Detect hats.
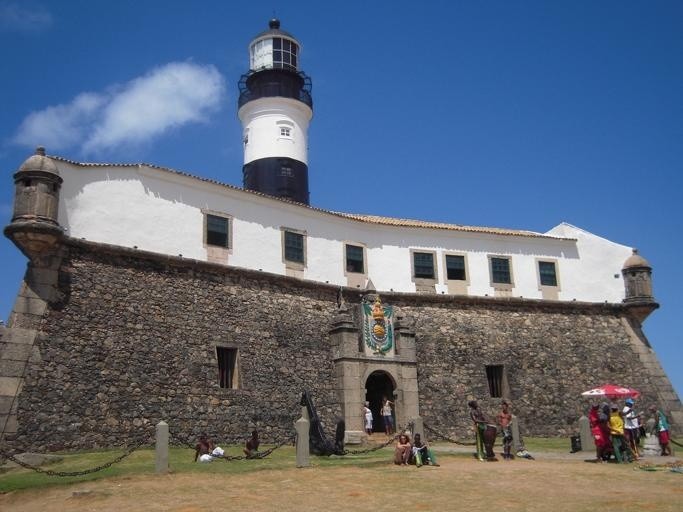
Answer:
[593,399,635,411]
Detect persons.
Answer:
[411,433,440,467]
[193,436,227,464]
[587,398,647,464]
[467,399,490,463]
[648,404,673,456]
[240,430,260,459]
[332,411,345,452]
[361,400,373,435]
[392,434,412,466]
[516,433,535,460]
[379,395,395,435]
[496,401,513,461]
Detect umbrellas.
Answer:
[579,382,641,402]
